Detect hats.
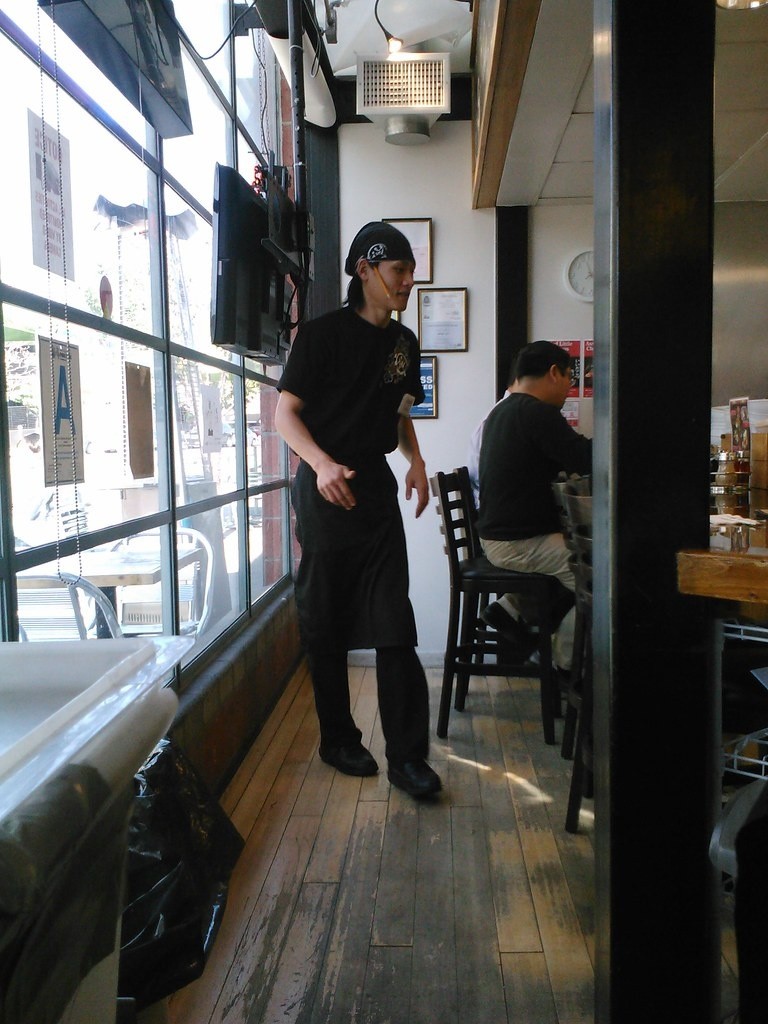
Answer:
[344,222,416,276]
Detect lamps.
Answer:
[375,0,403,53]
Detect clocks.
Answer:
[563,250,594,303]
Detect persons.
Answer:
[275,221,441,801]
[467,340,593,666]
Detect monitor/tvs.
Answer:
[209,163,291,367]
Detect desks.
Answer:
[20,545,203,641]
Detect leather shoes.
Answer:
[318,742,379,776]
[388,759,443,797]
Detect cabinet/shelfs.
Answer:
[712,605,768,955]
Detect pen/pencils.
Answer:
[373,266,391,298]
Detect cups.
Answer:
[710,525,732,554]
[731,525,750,553]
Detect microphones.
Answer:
[373,266,391,299]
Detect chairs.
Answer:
[18,526,215,641]
[557,475,595,835]
[428,468,564,742]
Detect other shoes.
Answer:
[483,601,536,649]
